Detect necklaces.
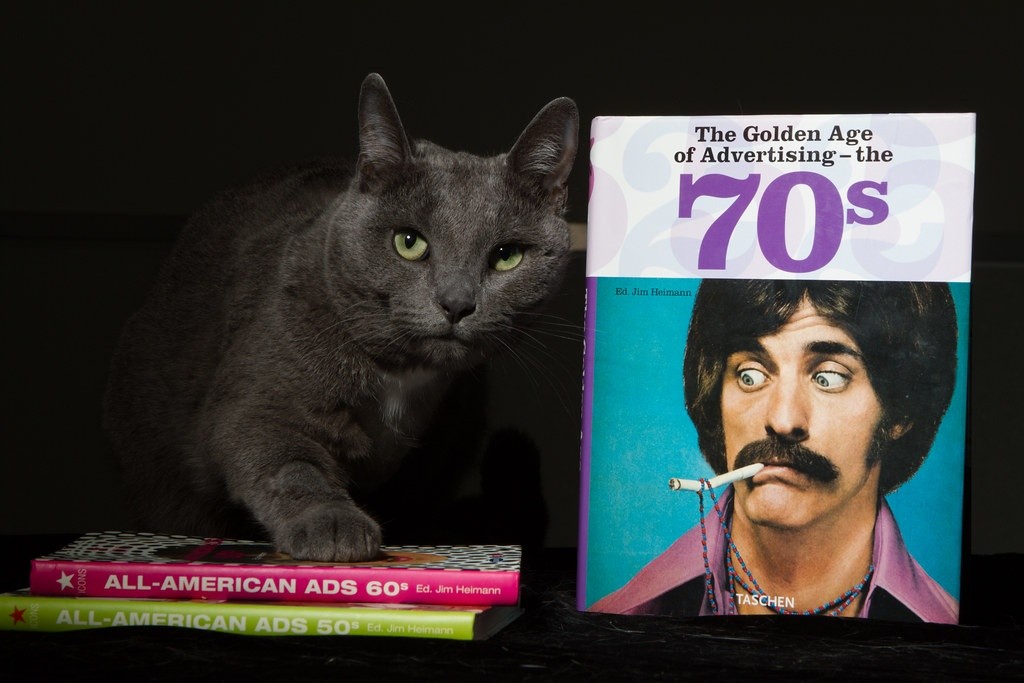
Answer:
[698,478,875,615]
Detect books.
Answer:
[576,112,976,624]
[29,526,521,605]
[0,587,525,642]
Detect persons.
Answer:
[590,279,957,624]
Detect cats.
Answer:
[0,70,579,563]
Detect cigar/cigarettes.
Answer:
[669,464,764,493]
